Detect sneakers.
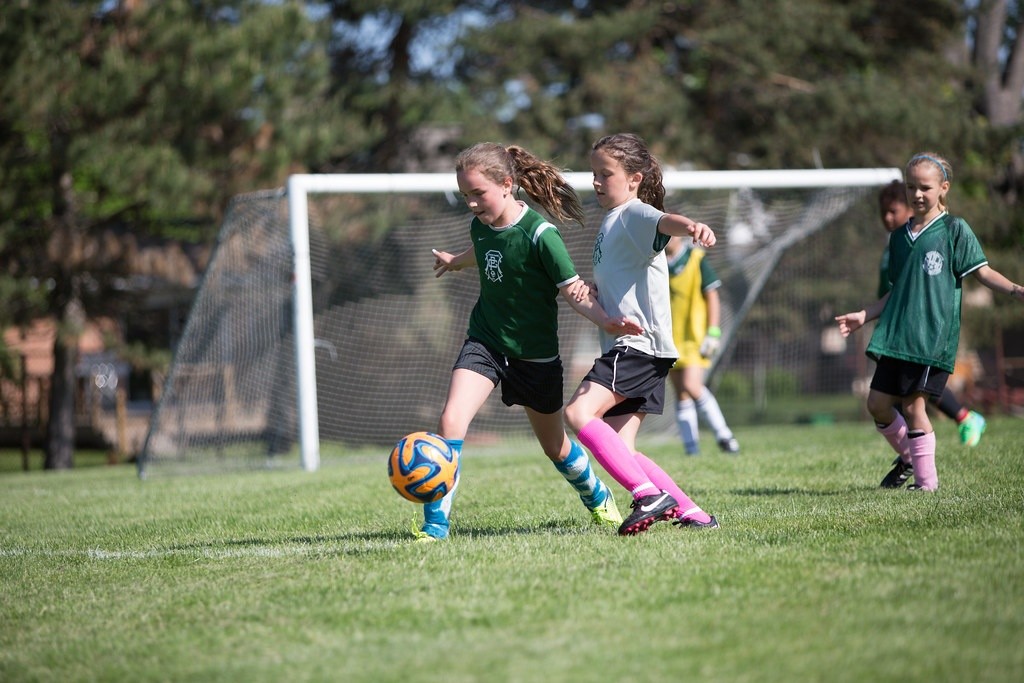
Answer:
[588,485,623,528]
[410,508,451,545]
[906,484,932,493]
[958,410,987,447]
[618,489,680,535]
[671,515,721,531]
[880,457,914,490]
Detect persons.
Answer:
[833,151,1024,490]
[875,178,986,448]
[407,141,646,543]
[661,232,740,458]
[561,133,722,536]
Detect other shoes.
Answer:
[718,436,740,453]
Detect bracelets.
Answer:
[709,326,721,336]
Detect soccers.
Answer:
[385,431,460,502]
